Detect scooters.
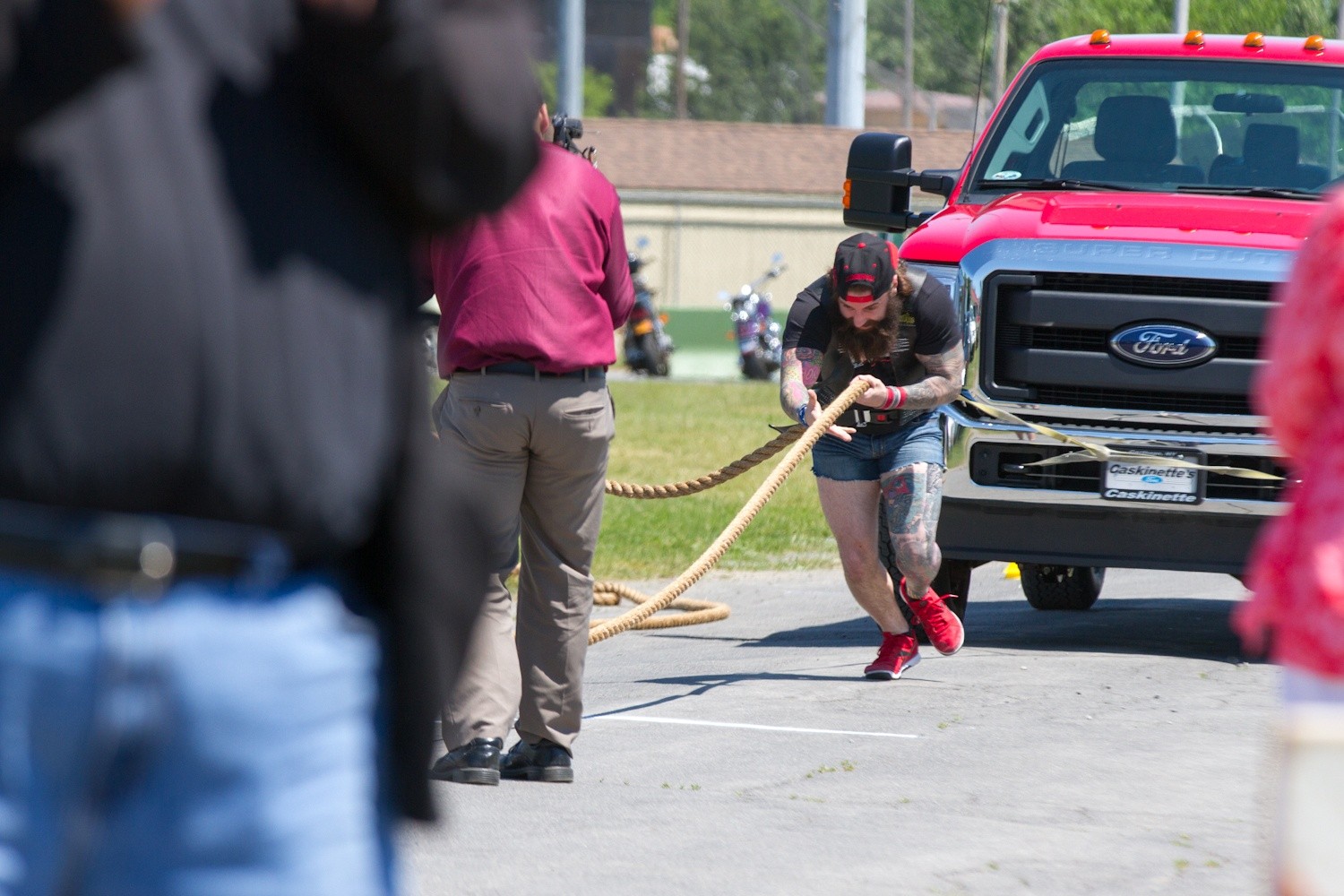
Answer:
[617,237,676,377]
[716,250,791,382]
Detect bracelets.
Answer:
[796,404,809,428]
[876,386,906,411]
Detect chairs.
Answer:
[1067,94,1204,184]
[1208,123,1329,189]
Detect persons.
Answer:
[779,233,965,680]
[1233,189,1344,895]
[427,105,636,784]
[0,1,542,896]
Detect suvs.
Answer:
[840,26,1344,645]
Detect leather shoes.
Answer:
[432,737,501,784]
[500,739,573,781]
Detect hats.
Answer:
[831,233,898,303]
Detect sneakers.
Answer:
[865,629,920,679]
[900,577,964,656]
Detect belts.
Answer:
[1,536,317,594]
[456,362,605,380]
